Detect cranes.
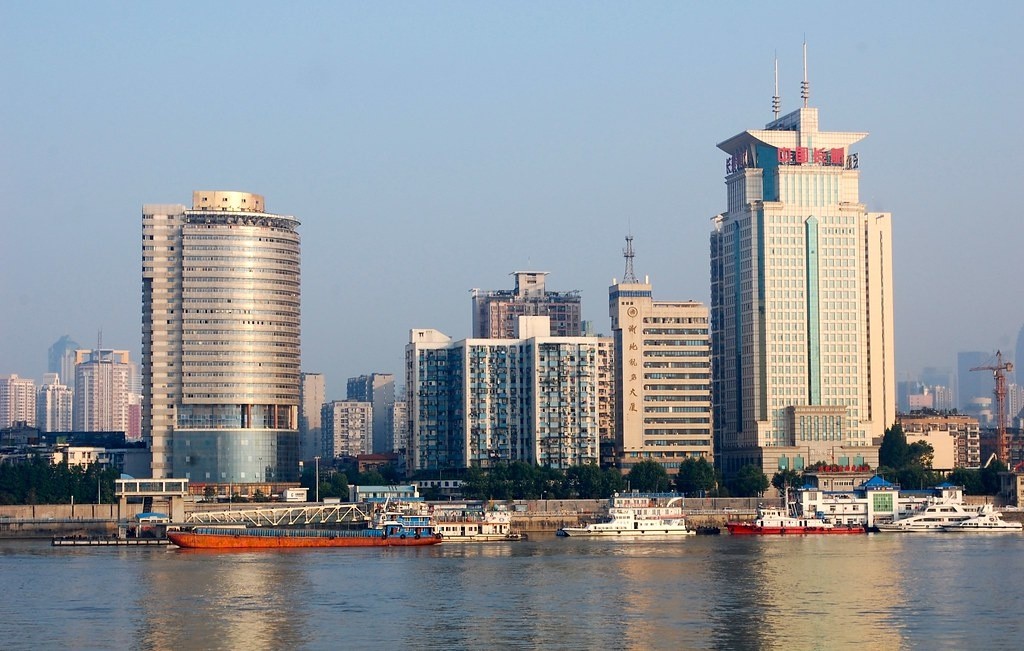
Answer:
[968,348,1015,467]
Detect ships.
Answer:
[722,483,867,535]
[871,502,981,533]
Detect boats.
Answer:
[555,514,696,537]
[171,502,446,547]
[940,503,1022,533]
[436,533,521,542]
